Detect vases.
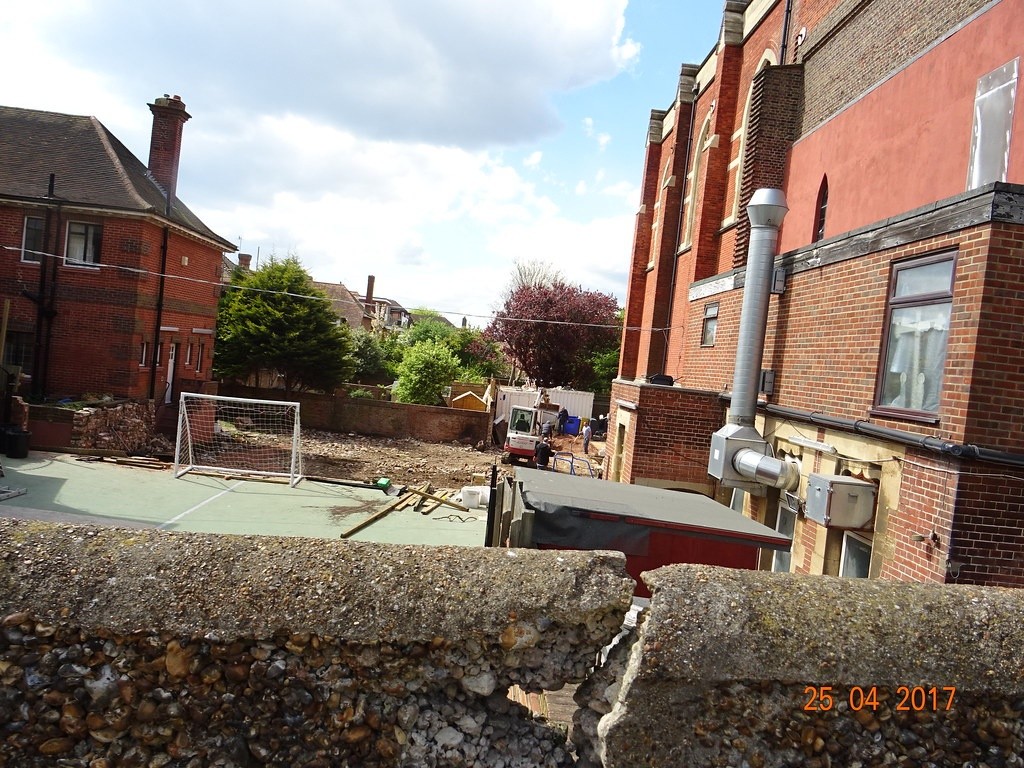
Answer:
[6,431,32,458]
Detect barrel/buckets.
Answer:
[478,486,490,504]
[461,485,480,509]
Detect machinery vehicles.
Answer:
[500,387,561,470]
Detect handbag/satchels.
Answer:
[533,456,539,462]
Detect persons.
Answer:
[579,421,591,454]
[534,438,556,470]
[556,407,570,436]
[515,414,530,433]
[542,421,553,440]
